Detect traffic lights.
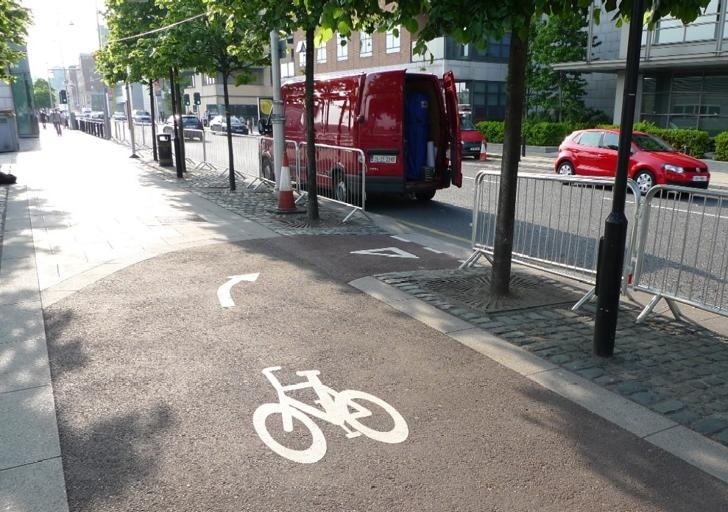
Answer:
[61,90,67,103]
[193,92,201,106]
[184,94,190,106]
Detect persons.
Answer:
[203,110,211,127]
[40,107,69,137]
[248,118,253,134]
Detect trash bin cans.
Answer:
[157,134,173,166]
[0,110,19,152]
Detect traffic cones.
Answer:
[479,139,488,161]
[264,152,308,214]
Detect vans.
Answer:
[257,68,462,200]
[457,116,486,158]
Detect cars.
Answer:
[554,127,709,197]
[114,111,127,120]
[162,114,205,140]
[132,109,152,127]
[209,115,249,135]
